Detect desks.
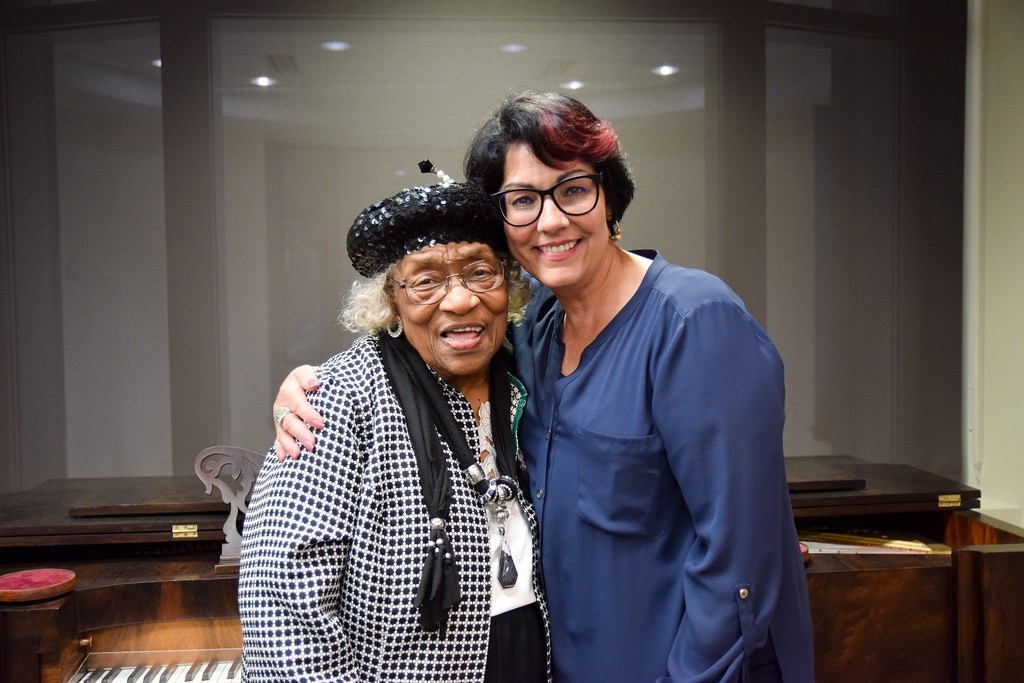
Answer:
[0,454,984,566]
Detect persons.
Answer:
[273,82,816,683]
[238,156,555,683]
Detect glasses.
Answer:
[390,259,507,305]
[491,171,604,227]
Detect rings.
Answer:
[276,406,292,427]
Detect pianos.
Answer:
[1,454,1023,683]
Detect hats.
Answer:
[346,158,510,279]
